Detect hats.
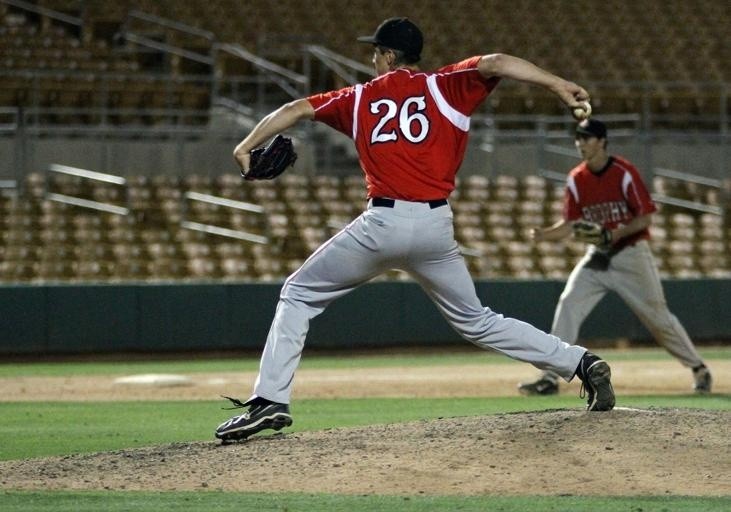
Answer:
[569,117,607,141]
[356,17,423,56]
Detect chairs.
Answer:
[0,0,731,280]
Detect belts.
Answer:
[372,198,448,209]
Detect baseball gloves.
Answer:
[241,134,297,181]
[572,218,613,247]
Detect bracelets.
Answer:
[601,227,612,245]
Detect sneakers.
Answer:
[518,376,559,396]
[215,394,294,441]
[576,351,616,412]
[691,364,714,391]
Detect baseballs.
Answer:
[574,100,591,118]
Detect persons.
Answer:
[215,16,617,445]
[515,117,714,396]
